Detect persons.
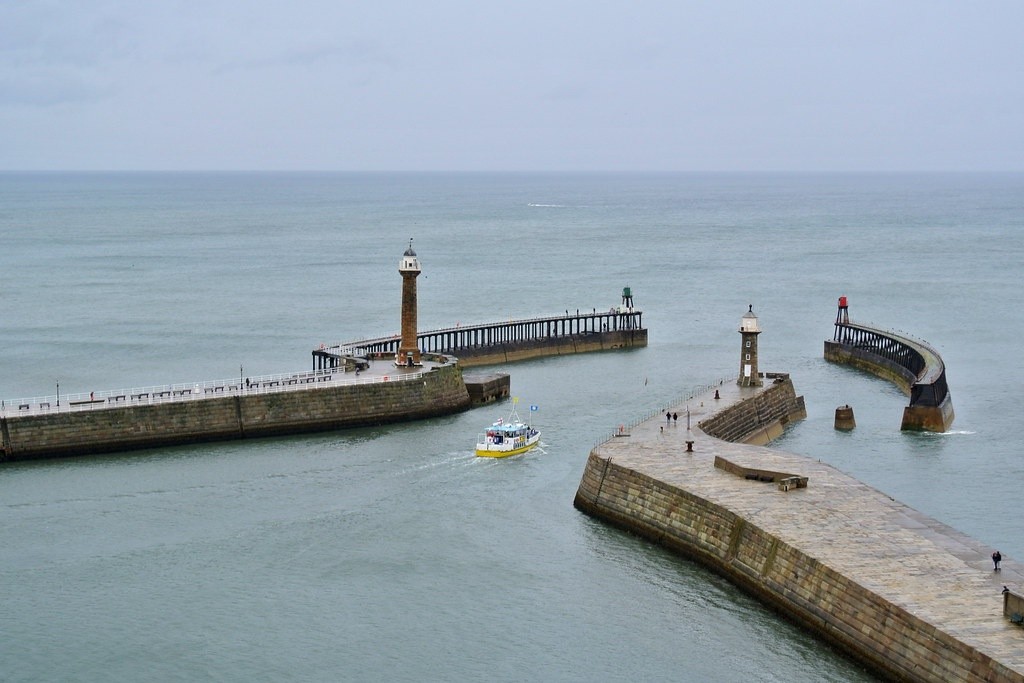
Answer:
[90,391,94,401]
[603,323,606,331]
[553,328,556,334]
[246,378,249,386]
[666,412,671,421]
[673,413,678,420]
[1,400,5,410]
[992,551,1002,571]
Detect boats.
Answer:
[475,397,541,458]
